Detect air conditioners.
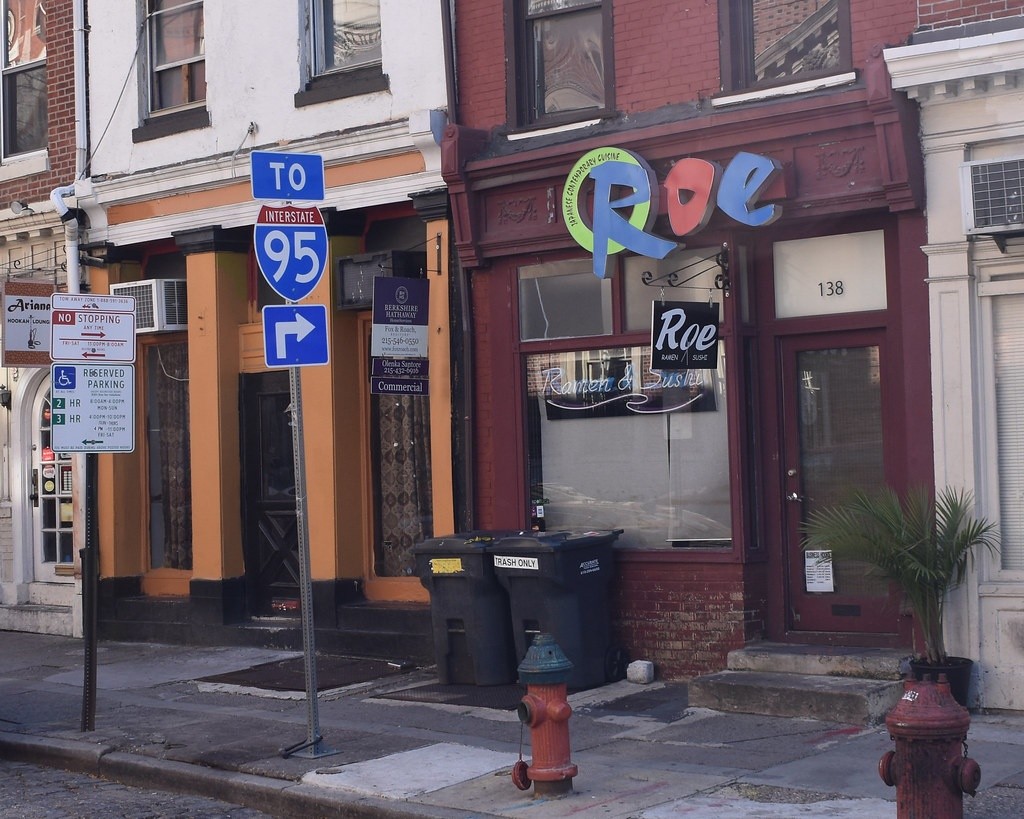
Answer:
[335,251,424,313]
[109,279,187,336]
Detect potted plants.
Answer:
[795,484,1011,706]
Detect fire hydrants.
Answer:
[511,633,579,798]
[878,673,982,818]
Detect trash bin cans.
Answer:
[409,530,515,690]
[487,526,632,693]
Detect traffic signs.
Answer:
[49,310,137,364]
[49,363,136,455]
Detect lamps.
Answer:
[0,384,12,408]
[11,199,36,214]
